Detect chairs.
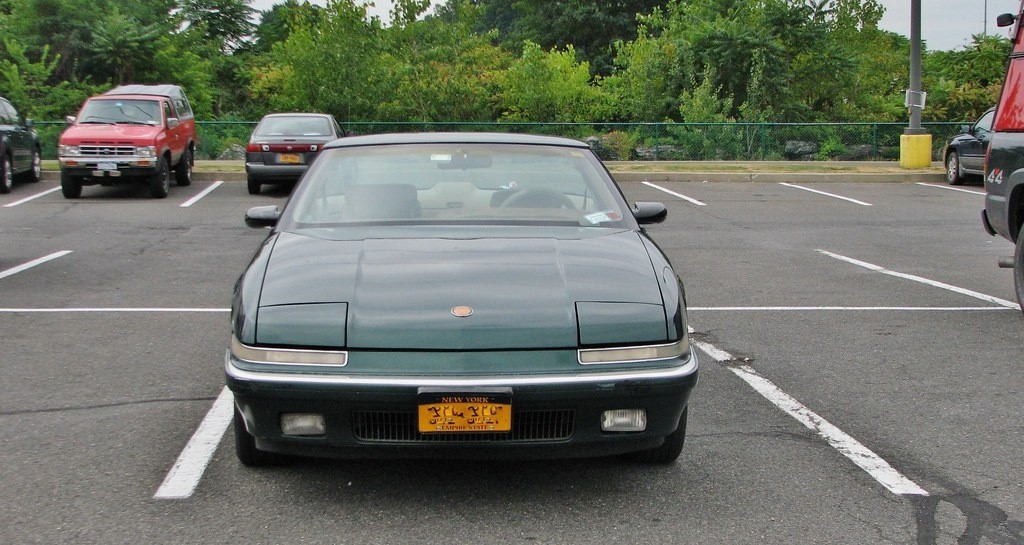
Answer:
[490,188,566,208]
[341,181,418,221]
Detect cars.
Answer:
[0,97,41,194]
[246,113,357,194]
[943,106,996,185]
[224,132,700,467]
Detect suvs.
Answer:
[58,85,197,198]
[980,1,1023,312]
